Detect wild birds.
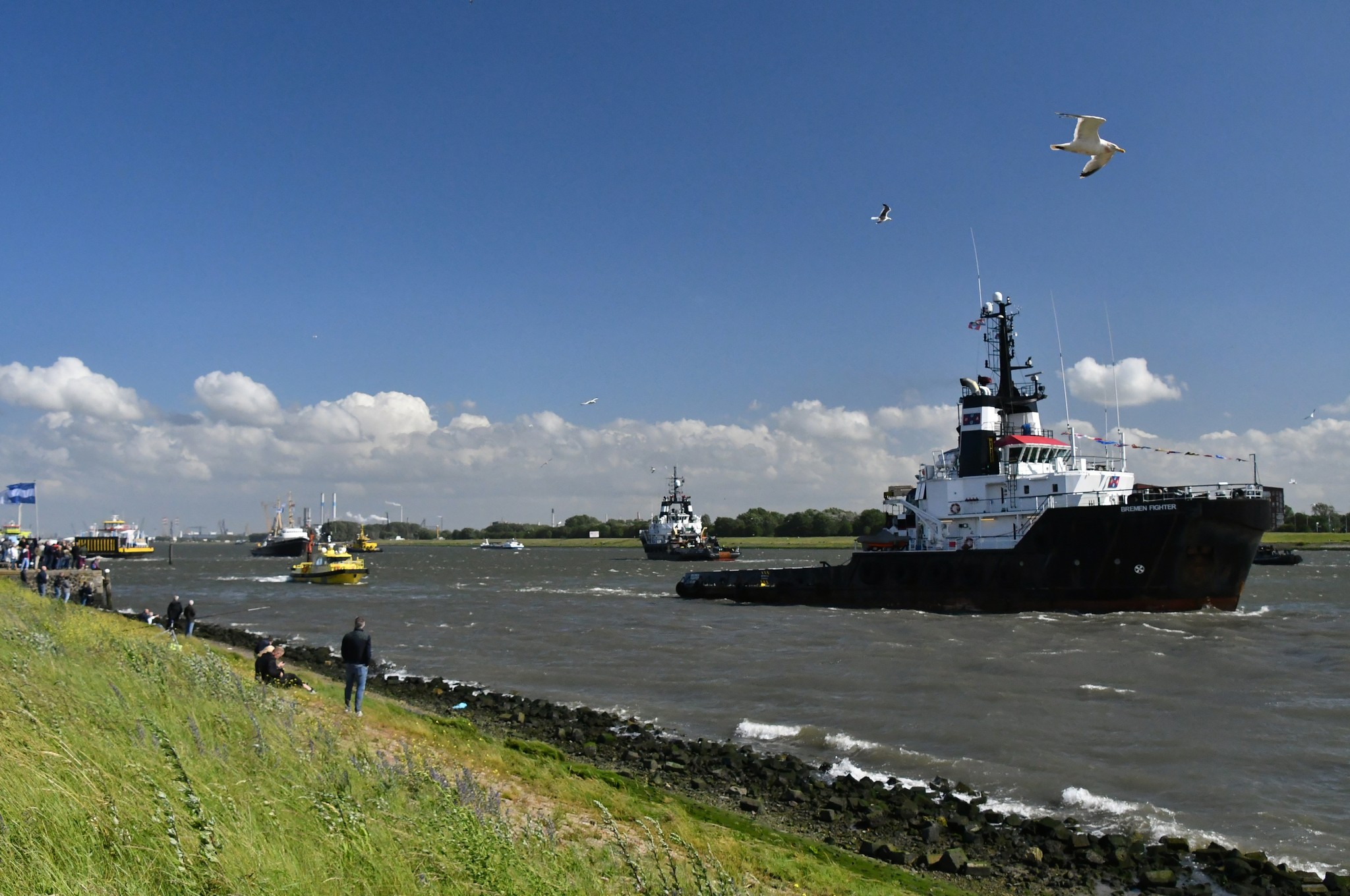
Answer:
[664,465,669,469]
[649,465,656,473]
[1303,408,1316,420]
[540,458,552,468]
[1049,110,1127,180]
[1288,478,1298,485]
[870,201,893,225]
[580,397,599,406]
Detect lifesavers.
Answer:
[965,538,974,548]
[950,503,961,514]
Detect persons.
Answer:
[80,581,93,606]
[37,566,49,598]
[20,567,28,588]
[140,608,161,624]
[91,555,106,579]
[184,599,197,637]
[166,595,183,630]
[341,616,373,718]
[254,637,320,695]
[0,537,39,571]
[42,540,88,570]
[62,576,73,603]
[53,572,64,600]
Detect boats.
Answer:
[674,283,1275,615]
[1252,544,1303,565]
[317,542,352,563]
[72,514,154,560]
[638,463,742,562]
[856,534,912,550]
[343,522,384,553]
[479,538,525,550]
[288,555,370,585]
[249,491,312,557]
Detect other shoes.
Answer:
[356,711,363,717]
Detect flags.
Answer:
[1061,432,1249,462]
[976,319,986,325]
[6,483,36,504]
[968,322,980,330]
[0,490,10,504]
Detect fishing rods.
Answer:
[122,606,270,632]
